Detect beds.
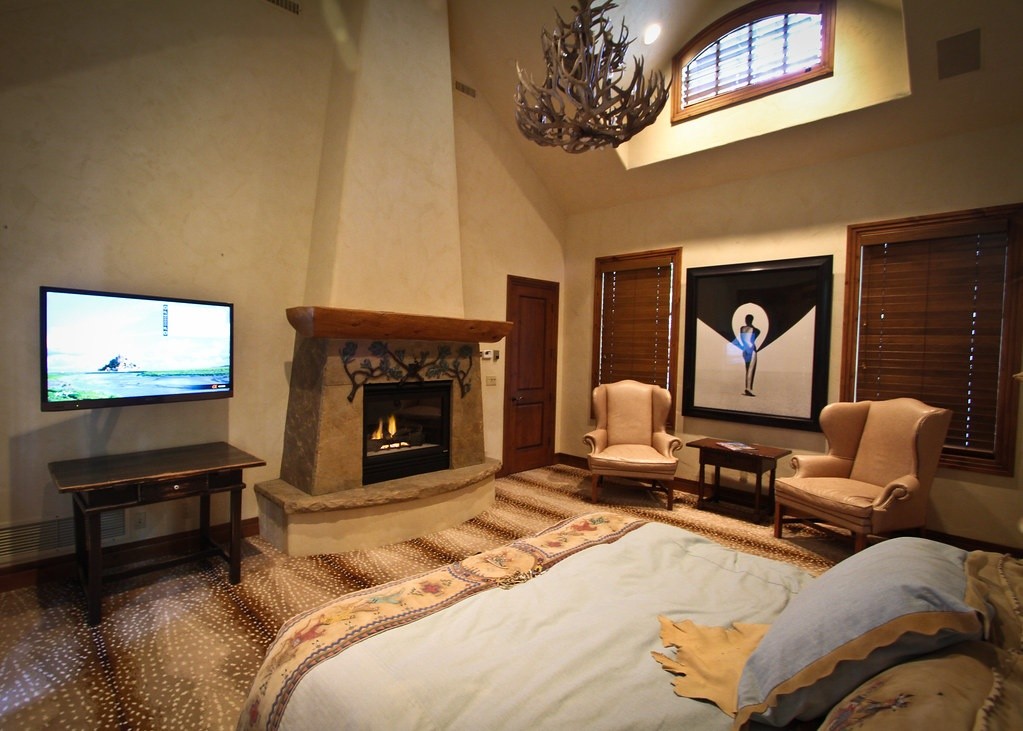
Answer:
[234,507,1023,731]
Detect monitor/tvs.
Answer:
[39,285,234,412]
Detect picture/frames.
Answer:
[681,251,836,431]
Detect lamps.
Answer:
[511,1,675,153]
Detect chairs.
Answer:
[774,395,951,557]
[580,379,680,512]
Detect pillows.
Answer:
[734,535,985,729]
[814,651,1005,731]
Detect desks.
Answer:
[48,441,267,628]
[685,436,792,524]
